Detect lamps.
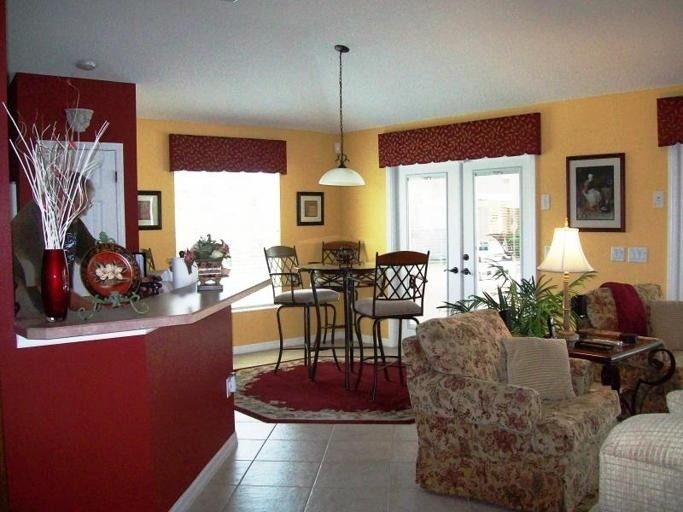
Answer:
[318,42,369,188]
[535,227,595,356]
[62,106,95,134]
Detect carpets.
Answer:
[231,356,416,425]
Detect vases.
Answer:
[39,248,70,324]
[192,257,225,291]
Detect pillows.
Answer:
[641,299,682,351]
[499,335,576,403]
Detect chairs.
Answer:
[599,381,683,511]
[352,247,437,399]
[140,247,155,274]
[317,236,366,376]
[400,306,624,512]
[570,279,682,415]
[261,245,346,383]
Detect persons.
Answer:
[10,171,162,312]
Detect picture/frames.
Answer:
[565,152,626,233]
[137,190,162,230]
[296,191,324,227]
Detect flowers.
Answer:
[182,233,228,276]
[89,259,130,286]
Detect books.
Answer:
[574,337,622,352]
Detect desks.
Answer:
[291,263,397,390]
[544,330,663,419]
[539,321,678,422]
